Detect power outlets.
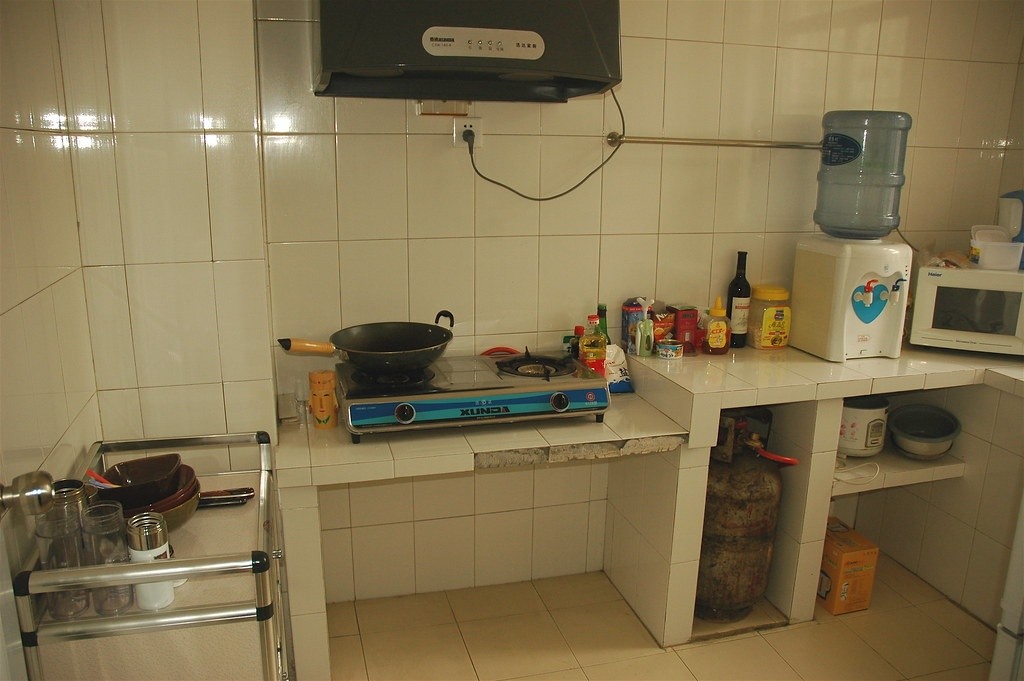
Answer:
[453,116,484,150]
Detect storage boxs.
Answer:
[969,241,1024,271]
[815,518,880,614]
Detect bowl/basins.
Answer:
[123,464,202,533]
[888,402,964,455]
[98,454,182,507]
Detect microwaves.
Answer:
[908,266,1024,356]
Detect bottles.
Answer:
[702,296,733,355]
[726,251,751,348]
[682,332,695,354]
[579,315,608,378]
[748,284,792,350]
[591,304,612,346]
[813,110,911,239]
[570,326,585,358]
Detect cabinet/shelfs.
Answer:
[12,430,291,681]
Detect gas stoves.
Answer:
[333,346,610,444]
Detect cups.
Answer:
[34,506,91,621]
[49,479,89,510]
[126,512,174,610]
[81,501,135,616]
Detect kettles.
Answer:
[998,188,1024,269]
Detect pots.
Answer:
[277,310,454,375]
[835,395,891,459]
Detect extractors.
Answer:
[308,0,622,104]
